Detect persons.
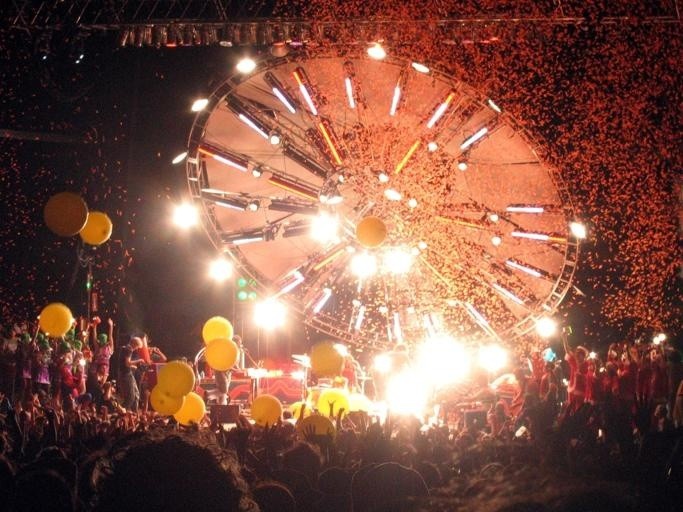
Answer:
[1,311,683,510]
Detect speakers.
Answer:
[464,409,492,432]
[208,404,243,423]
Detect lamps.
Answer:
[116,0,507,58]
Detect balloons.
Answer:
[354,217,387,245]
[39,192,349,441]
